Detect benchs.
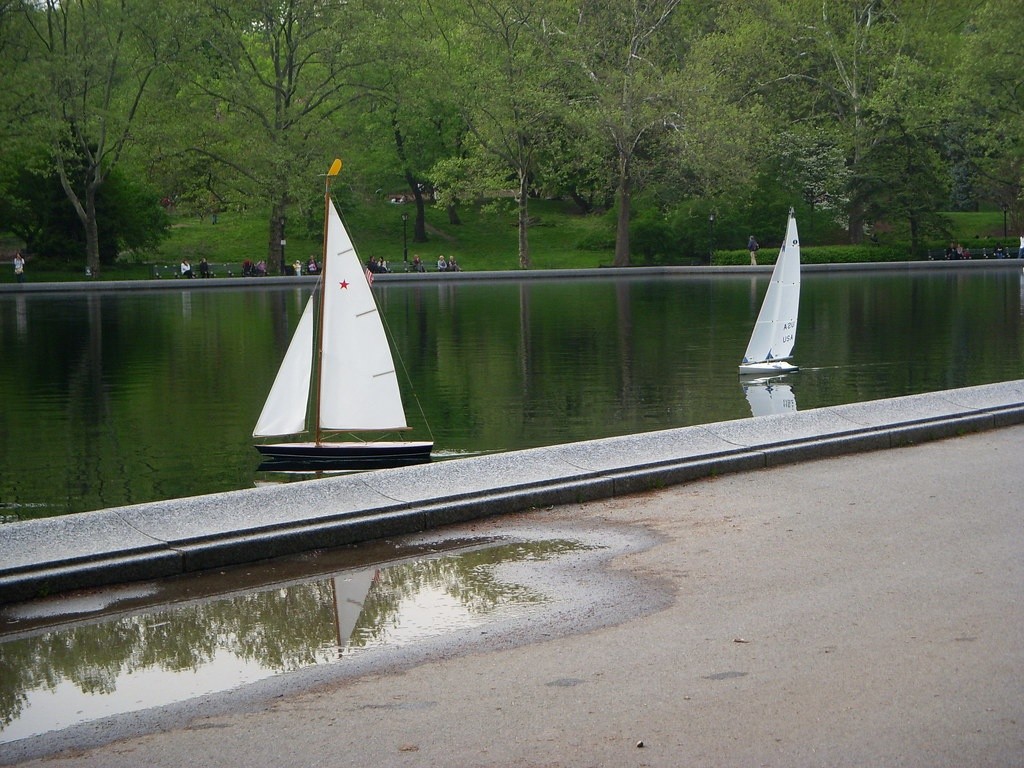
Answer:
[150,262,244,278]
[928,246,1019,261]
[285,264,323,276]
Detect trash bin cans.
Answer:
[285,265,294,275]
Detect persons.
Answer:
[946,233,1024,259]
[14,253,25,281]
[748,235,756,265]
[181,255,463,278]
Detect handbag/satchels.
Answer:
[15,268,23,274]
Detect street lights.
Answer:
[709,208,718,265]
[400,213,410,266]
[277,211,290,276]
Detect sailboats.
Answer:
[738,374,797,419]
[251,173,435,462]
[739,205,804,375]
[255,458,408,486]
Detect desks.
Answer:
[365,261,440,273]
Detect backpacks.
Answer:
[752,240,759,251]
